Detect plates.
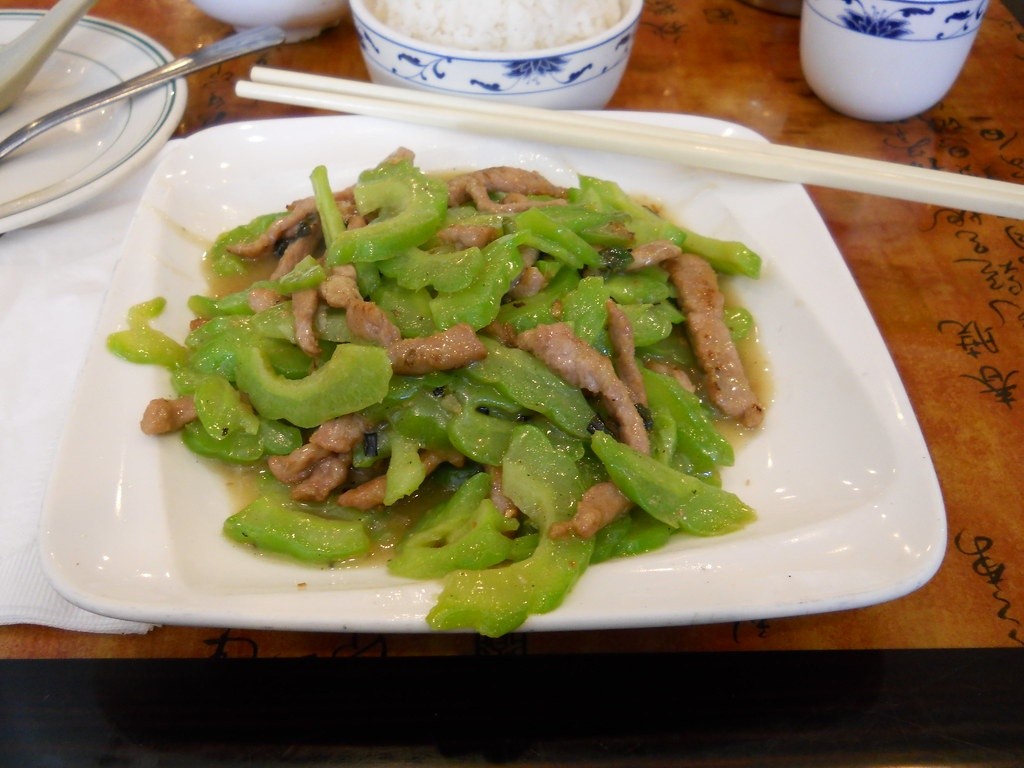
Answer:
[38,110,950,632]
[0,8,190,234]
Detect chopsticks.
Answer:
[235,65,1024,221]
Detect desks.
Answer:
[0,1,1024,765]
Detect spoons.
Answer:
[0,0,100,114]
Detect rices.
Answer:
[361,0,632,52]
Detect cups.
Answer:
[799,0,991,122]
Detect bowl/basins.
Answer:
[349,0,644,110]
[191,0,348,44]
